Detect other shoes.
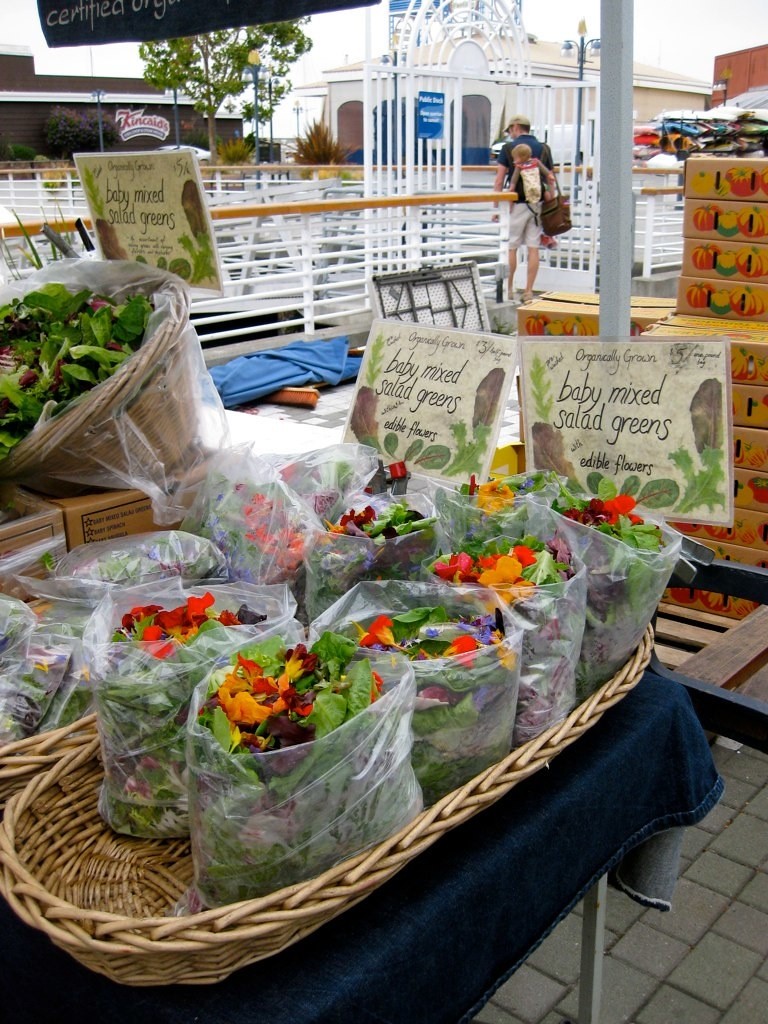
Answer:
[518,294,533,304]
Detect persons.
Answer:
[631,136,768,212]
[487,116,555,302]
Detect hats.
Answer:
[503,115,530,132]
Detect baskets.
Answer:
[0,266,198,496]
[0,620,655,987]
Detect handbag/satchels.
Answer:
[540,196,572,236]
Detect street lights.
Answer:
[268,77,280,181]
[561,19,602,206]
[292,101,303,138]
[242,51,268,188]
[92,88,107,152]
[381,51,398,192]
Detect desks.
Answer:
[0,642,726,1024]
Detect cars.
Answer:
[153,144,212,163]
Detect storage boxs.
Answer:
[40,456,210,550]
[514,150,768,625]
[0,478,68,604]
